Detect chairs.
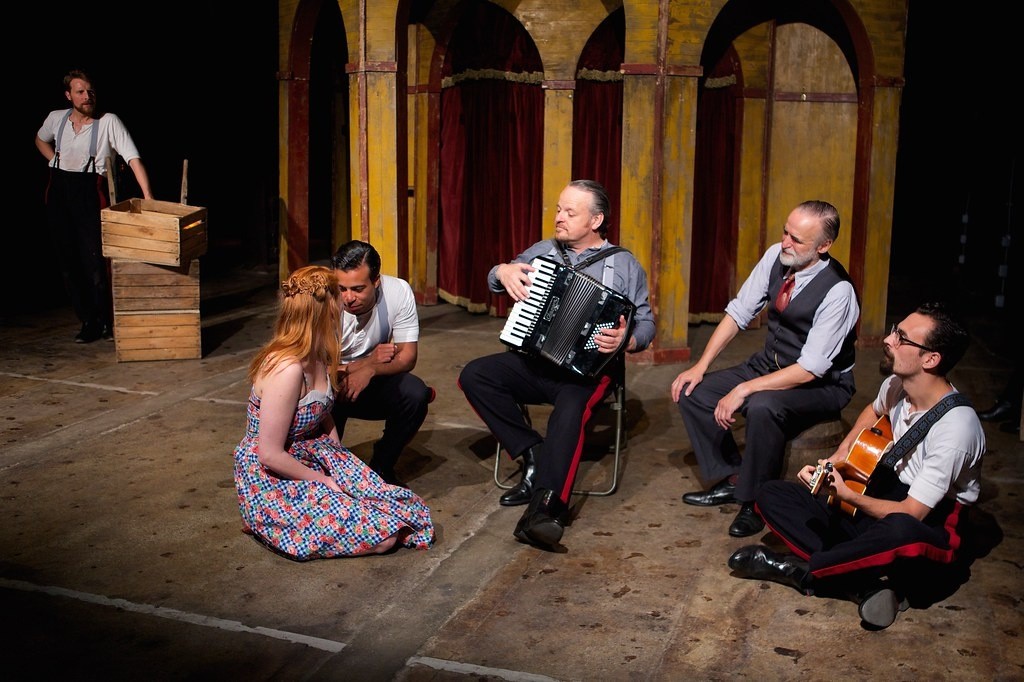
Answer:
[494,353,626,494]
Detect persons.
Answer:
[976,368,1024,434]
[670,200,860,536]
[330,240,429,489]
[457,180,656,547]
[725,299,988,630]
[35,70,154,343]
[232,265,435,562]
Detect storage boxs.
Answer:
[110,258,201,310]
[113,311,201,363]
[100,197,209,268]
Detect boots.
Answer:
[729,546,810,592]
[858,578,909,629]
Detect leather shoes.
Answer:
[977,399,1017,420]
[100,322,112,342]
[369,461,409,489]
[683,479,738,506]
[1000,418,1020,434]
[499,443,545,505]
[74,318,99,343]
[513,489,564,546]
[728,499,765,537]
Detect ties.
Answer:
[776,274,795,312]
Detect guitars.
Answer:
[806,412,897,519]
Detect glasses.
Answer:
[891,323,935,351]
